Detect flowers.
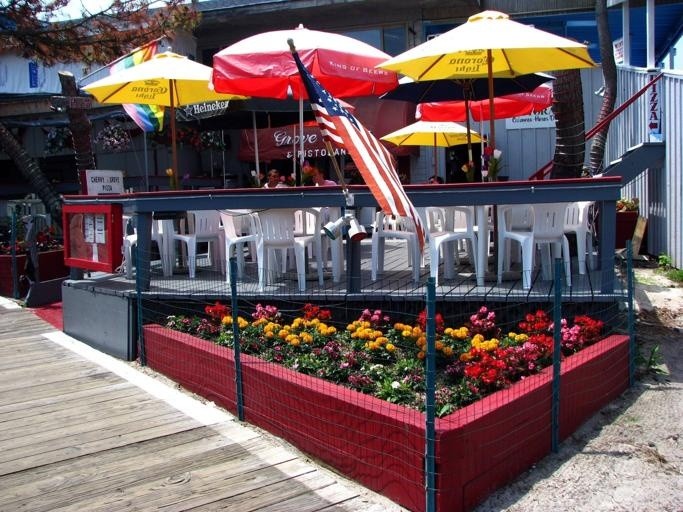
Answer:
[459,145,505,183]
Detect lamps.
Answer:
[323,187,374,250]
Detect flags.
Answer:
[290,53,425,255]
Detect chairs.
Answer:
[121,198,598,293]
